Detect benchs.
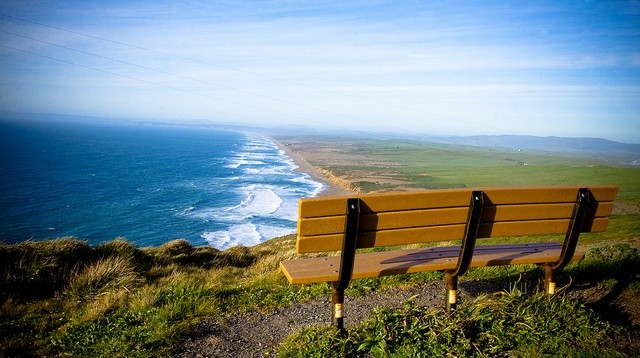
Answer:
[278,186,619,330]
